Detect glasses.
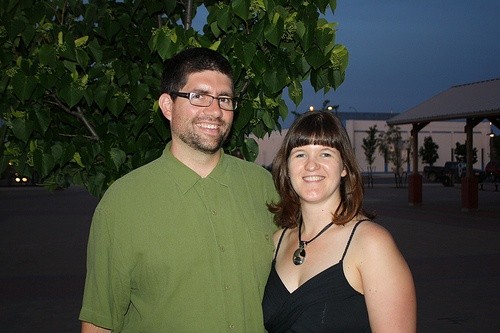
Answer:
[169,91,239,111]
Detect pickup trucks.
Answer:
[423,162,486,183]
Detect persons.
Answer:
[261,109,417,333]
[79,47,283,333]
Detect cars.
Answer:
[485,161,500,182]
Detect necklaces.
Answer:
[292,198,343,266]
